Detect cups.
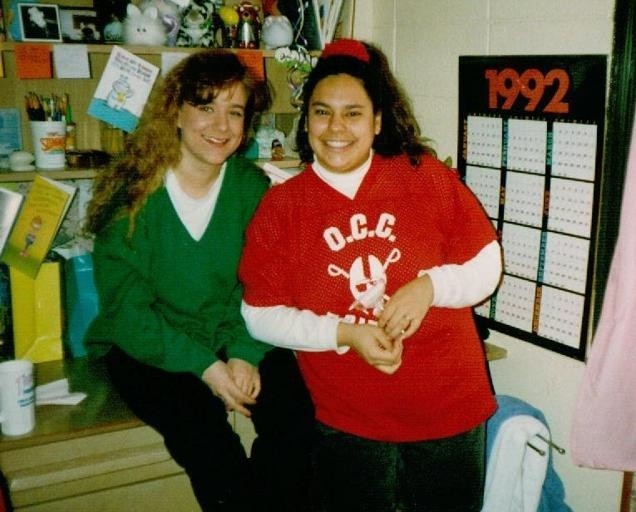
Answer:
[27,119,68,170]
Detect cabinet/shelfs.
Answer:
[0,0,505,512]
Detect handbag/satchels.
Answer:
[9,233,101,364]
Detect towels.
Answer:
[569,116,636,472]
[481,395,576,512]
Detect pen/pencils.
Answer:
[25,91,72,125]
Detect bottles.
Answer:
[0,361,36,436]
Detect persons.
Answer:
[83,49,321,508]
[238,38,503,512]
